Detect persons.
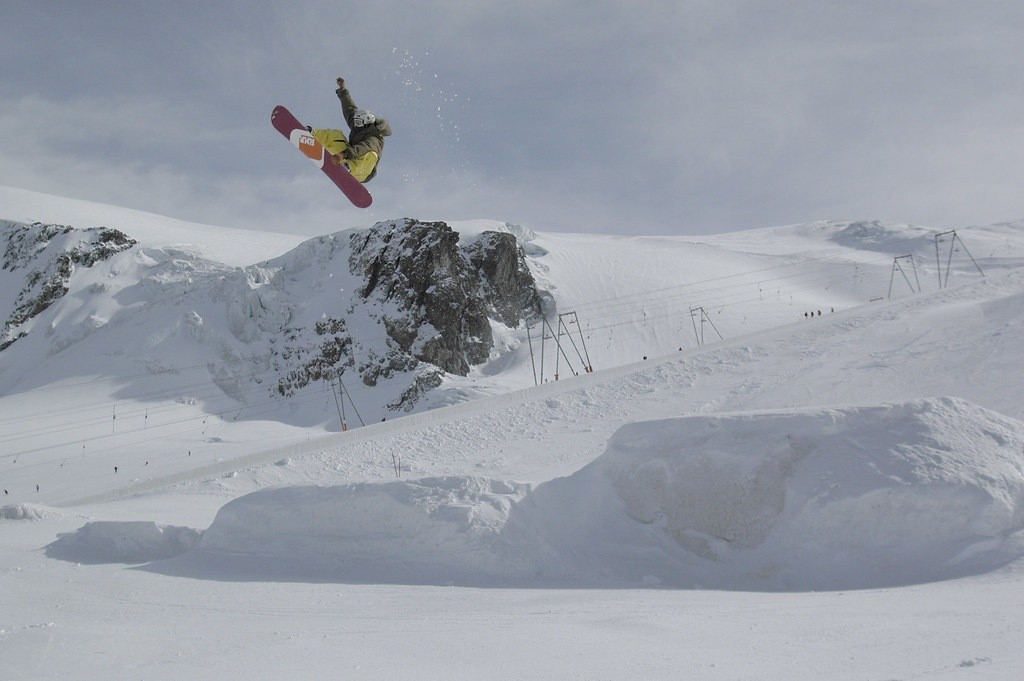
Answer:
[304,76,385,183]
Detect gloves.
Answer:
[331,151,347,165]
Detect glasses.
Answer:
[354,119,364,128]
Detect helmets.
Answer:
[353,111,376,129]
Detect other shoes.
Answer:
[343,164,350,172]
[305,125,312,133]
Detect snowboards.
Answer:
[270,105,374,209]
